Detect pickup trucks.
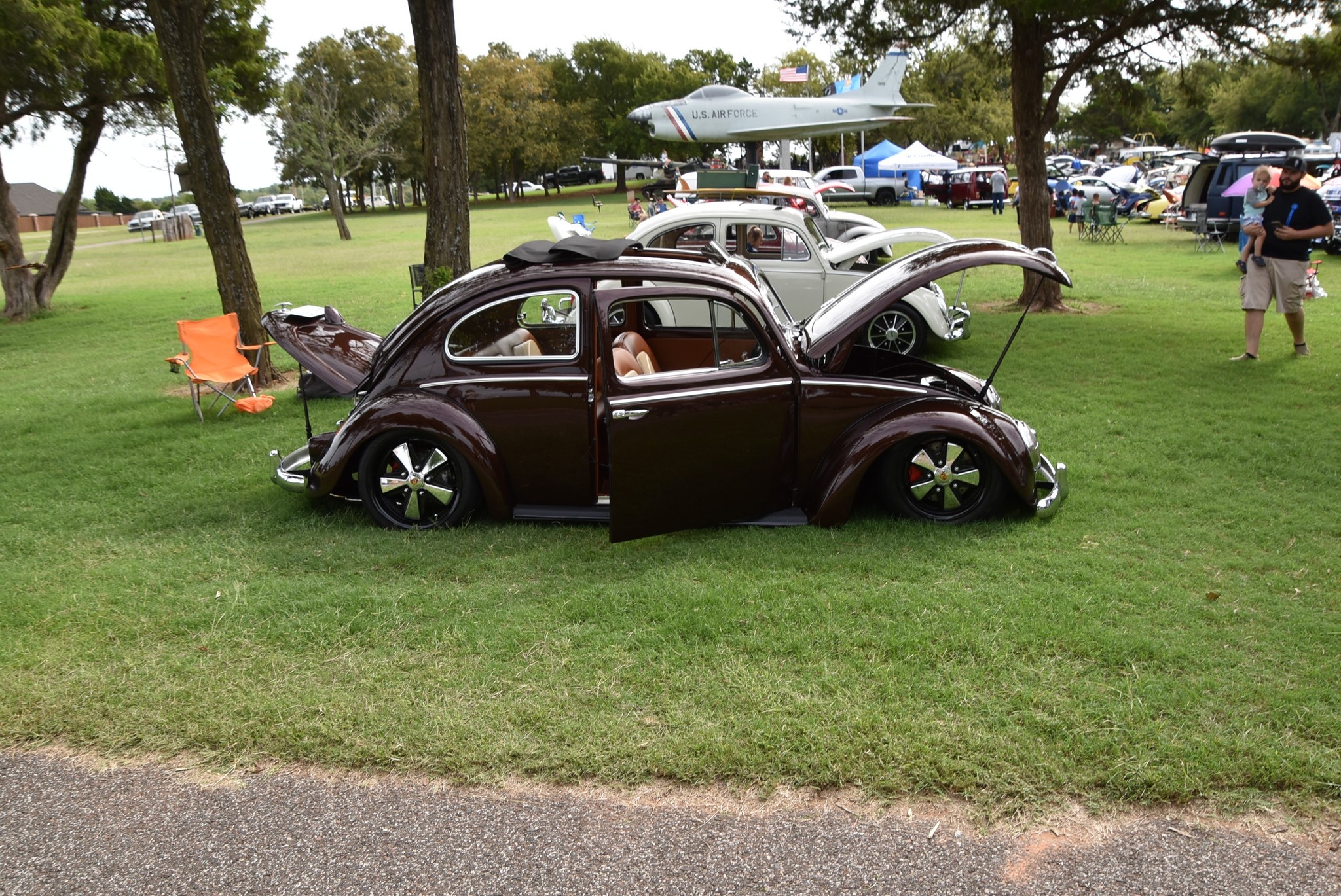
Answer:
[541,165,605,190]
[802,166,908,208]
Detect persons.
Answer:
[784,177,796,207]
[1046,186,1053,219]
[1334,152,1341,159]
[1153,140,1190,150]
[1044,145,1086,159]
[1132,162,1139,184]
[1068,189,1101,234]
[661,150,671,169]
[1228,156,1332,362]
[919,169,952,196]
[748,226,764,253]
[1012,186,1021,233]
[630,197,668,225]
[989,169,1007,215]
[902,172,917,198]
[1236,165,1276,273]
[1095,163,1105,177]
[1071,157,1082,171]
[674,166,682,186]
[754,172,773,204]
[1174,156,1182,162]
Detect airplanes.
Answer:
[626,41,936,143]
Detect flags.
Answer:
[779,65,809,82]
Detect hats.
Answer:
[1277,156,1307,172]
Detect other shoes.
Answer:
[1235,260,1247,274]
[1293,342,1310,357]
[1251,255,1266,268]
[1228,353,1260,361]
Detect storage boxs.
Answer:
[928,199,941,206]
[911,199,926,207]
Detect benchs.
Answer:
[592,194,603,213]
[453,327,545,356]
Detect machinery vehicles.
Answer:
[578,155,712,200]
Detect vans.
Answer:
[947,166,1011,210]
[758,170,836,204]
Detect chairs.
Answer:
[1158,200,1182,234]
[611,347,645,378]
[1090,199,1127,246]
[1079,199,1112,242]
[612,331,662,375]
[628,200,661,229]
[1306,260,1322,288]
[1192,210,1232,253]
[573,214,597,232]
[408,264,424,311]
[162,310,278,423]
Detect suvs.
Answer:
[321,190,359,211]
[364,195,396,208]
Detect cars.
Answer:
[127,193,304,233]
[540,181,972,356]
[501,181,543,194]
[1046,131,1341,255]
[260,235,1072,545]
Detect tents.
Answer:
[855,139,921,202]
[878,140,958,201]
